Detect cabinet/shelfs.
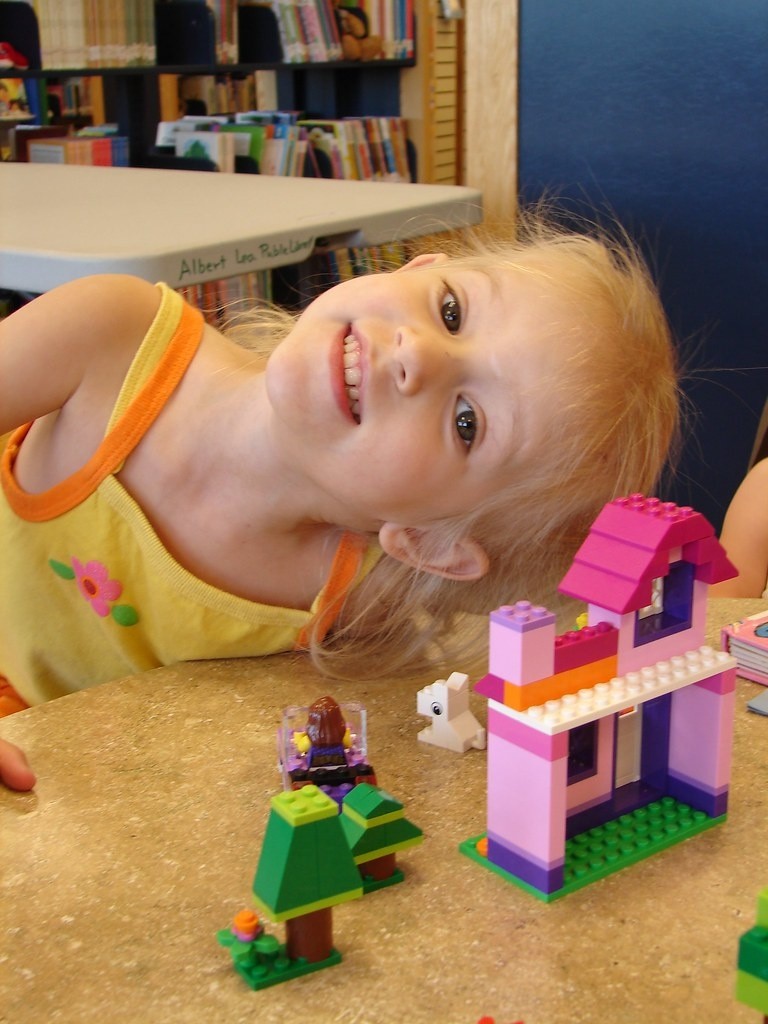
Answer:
[0,0,418,317]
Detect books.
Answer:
[0,0,418,332]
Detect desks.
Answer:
[0,161,485,294]
[0,597,768,1024]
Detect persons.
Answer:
[707,456,768,598]
[0,231,677,792]
